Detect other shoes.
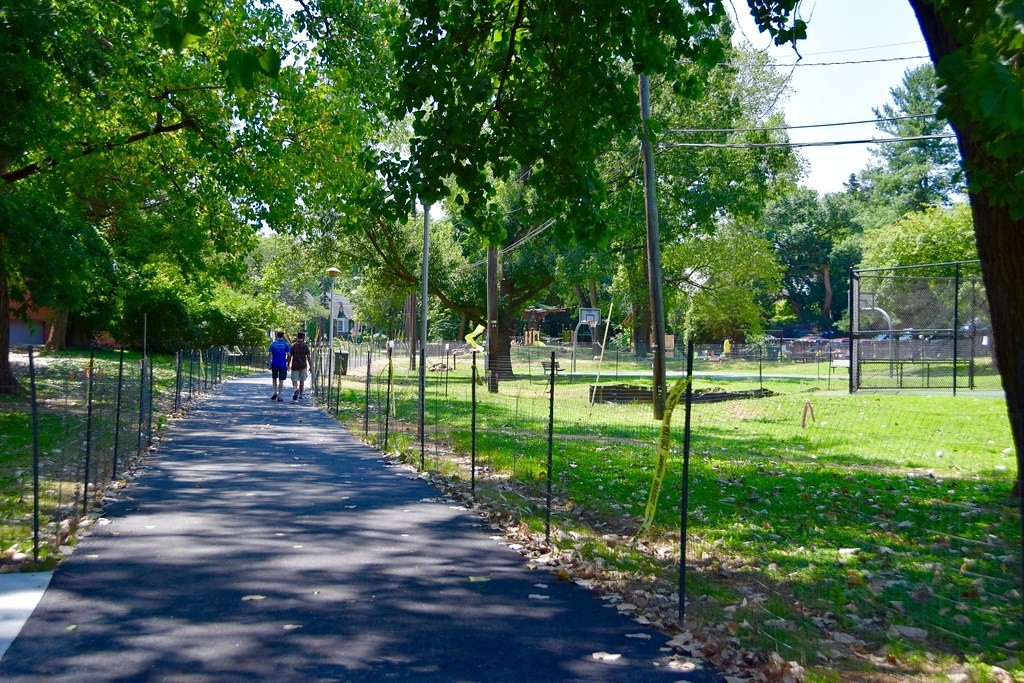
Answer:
[297,396,302,401]
[270,393,278,400]
[277,396,284,401]
[293,390,299,400]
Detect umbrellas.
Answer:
[796,336,878,344]
[745,334,778,342]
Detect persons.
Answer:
[290,333,314,400]
[724,337,732,353]
[809,344,814,352]
[268,331,291,401]
[386,338,394,357]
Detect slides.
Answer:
[464,324,486,352]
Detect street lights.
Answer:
[326,267,342,407]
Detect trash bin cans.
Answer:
[334,350,350,375]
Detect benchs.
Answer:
[542,361,566,374]
